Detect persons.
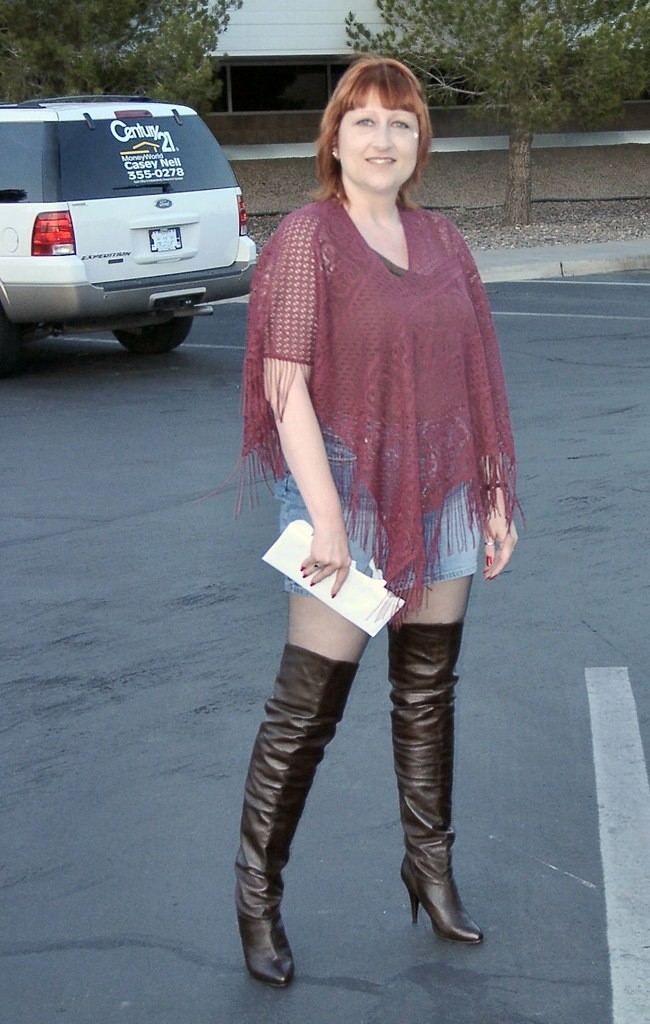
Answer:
[233,54,524,990]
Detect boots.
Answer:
[235,643,361,986]
[388,618,484,943]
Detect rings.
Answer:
[484,542,496,546]
[313,564,323,570]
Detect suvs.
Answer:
[0,94,259,377]
